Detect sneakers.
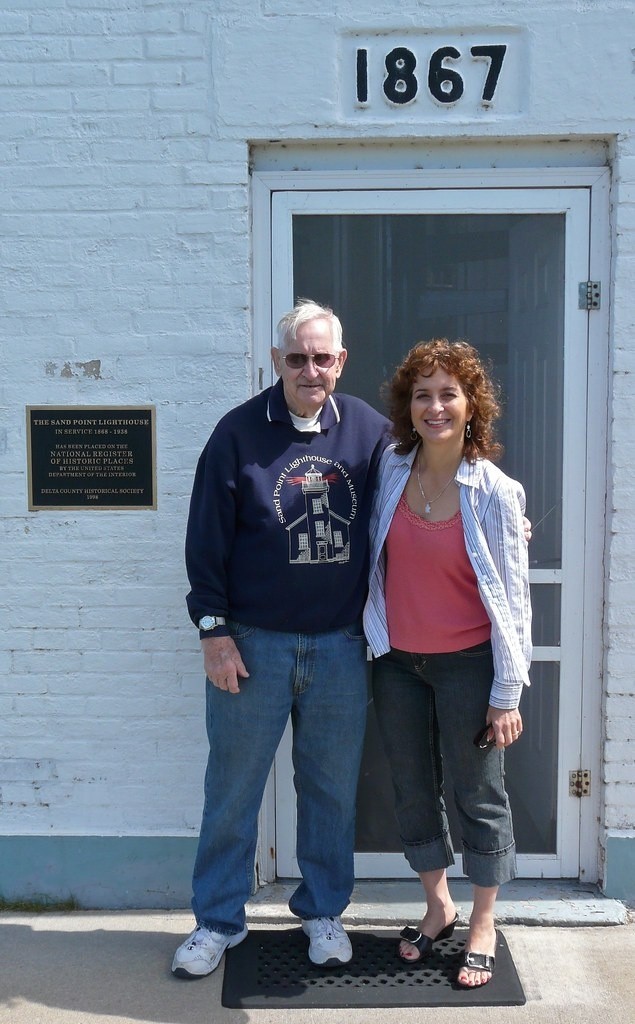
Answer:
[172,921,248,981]
[302,913,353,966]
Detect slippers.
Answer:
[456,928,498,988]
[399,911,459,963]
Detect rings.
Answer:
[512,731,519,736]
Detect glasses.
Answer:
[279,353,339,368]
[473,722,496,747]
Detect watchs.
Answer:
[199,616,225,632]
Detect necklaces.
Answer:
[417,453,455,514]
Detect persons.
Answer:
[172,304,532,980]
[362,340,534,989]
